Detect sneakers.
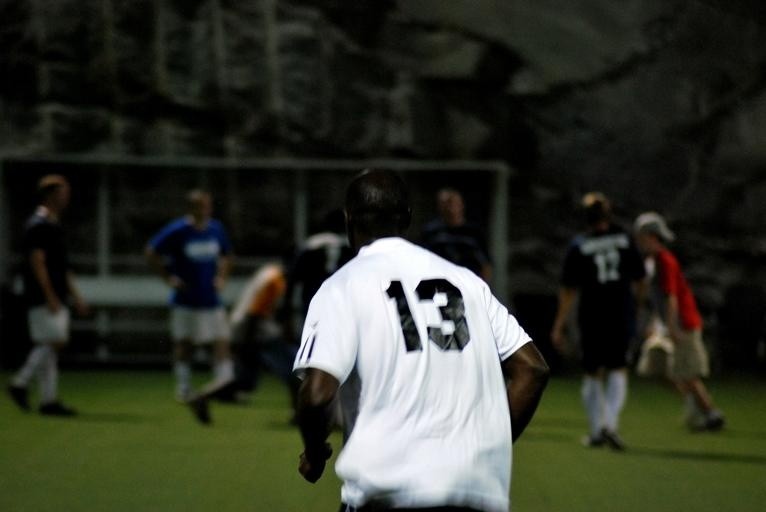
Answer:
[174,383,209,423]
[582,423,624,451]
[688,410,723,432]
[7,383,72,415]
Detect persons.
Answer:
[145,187,234,406]
[6,175,84,416]
[186,170,550,510]
[551,191,725,448]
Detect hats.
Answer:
[631,210,679,248]
